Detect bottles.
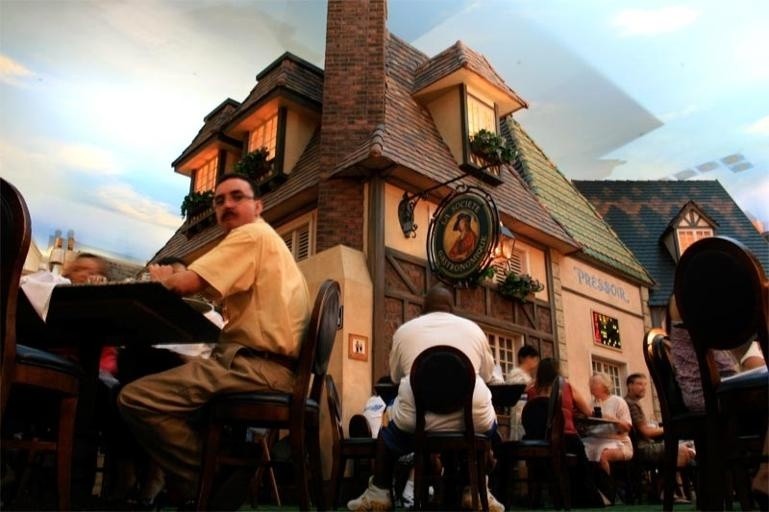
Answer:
[48,237,74,277]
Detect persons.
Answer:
[18,174,767,510]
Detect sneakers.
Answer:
[462,474,507,511]
[204,439,265,512]
[347,475,396,512]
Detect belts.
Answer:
[234,347,302,373]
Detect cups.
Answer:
[595,398,602,406]
[142,272,151,281]
[593,406,602,417]
[87,275,106,285]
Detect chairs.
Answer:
[0,178,85,512]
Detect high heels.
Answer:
[130,475,170,512]
